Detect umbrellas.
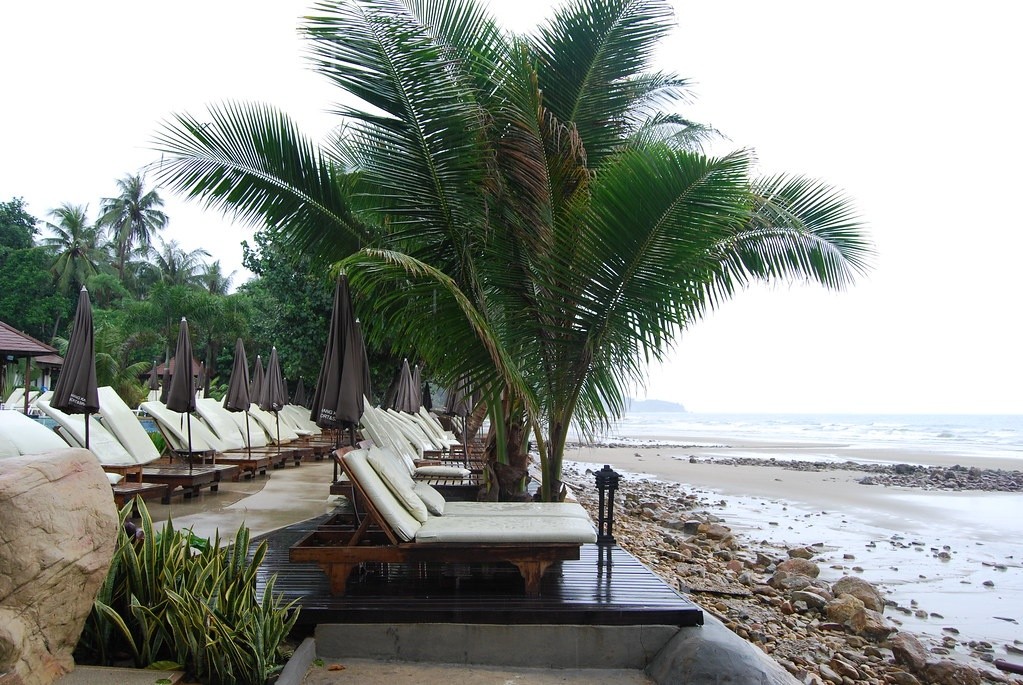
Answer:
[294,376,305,407]
[49,285,99,448]
[310,268,372,485]
[166,317,196,470]
[250,346,289,452]
[423,383,431,411]
[196,362,204,398]
[222,337,251,453]
[149,361,159,401]
[160,347,169,404]
[444,370,483,469]
[382,358,423,413]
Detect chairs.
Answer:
[0,386,599,596]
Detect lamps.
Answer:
[594,464,622,492]
